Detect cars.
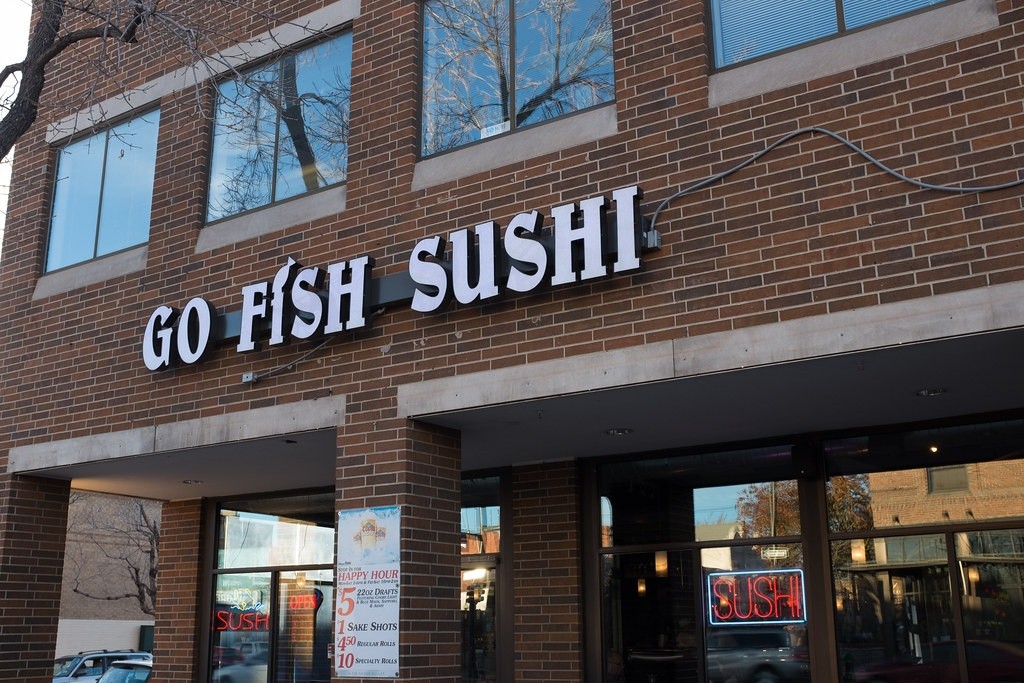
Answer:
[211,650,267,682]
[706,631,806,683]
[97,659,153,683]
[213,646,245,670]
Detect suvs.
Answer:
[52,648,153,683]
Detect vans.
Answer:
[241,643,269,659]
